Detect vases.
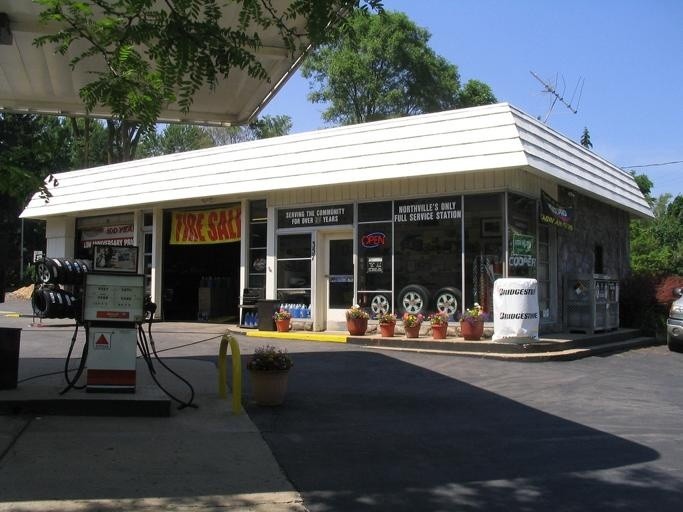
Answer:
[348,319,367,336]
[432,325,447,339]
[274,320,290,332]
[405,327,420,338]
[249,371,289,407]
[380,323,394,337]
[461,322,483,341]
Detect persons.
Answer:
[96,247,113,268]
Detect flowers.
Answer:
[428,312,449,326]
[457,303,490,327]
[402,313,425,327]
[346,307,368,320]
[246,345,293,370]
[272,308,291,319]
[378,313,398,325]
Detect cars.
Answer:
[665,287,682,352]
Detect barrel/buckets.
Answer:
[244,312,260,327]
[279,303,311,318]
